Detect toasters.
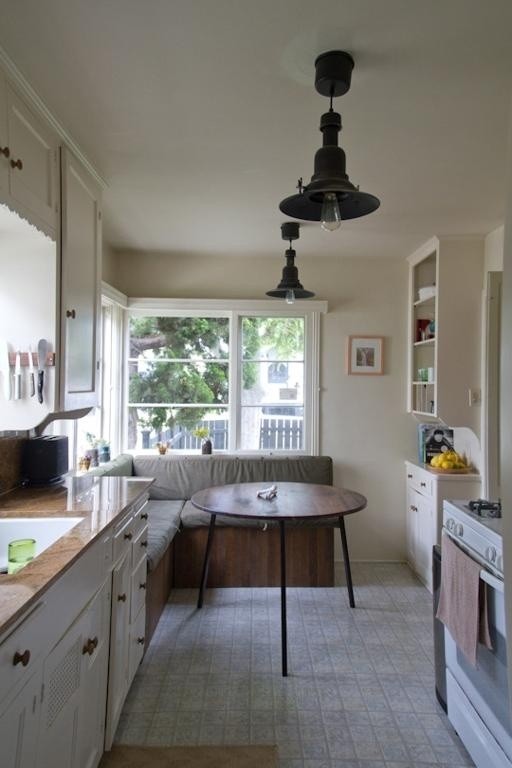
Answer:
[21,433,69,485]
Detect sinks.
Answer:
[0,515,85,584]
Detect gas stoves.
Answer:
[442,496,504,580]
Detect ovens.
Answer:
[442,542,511,767]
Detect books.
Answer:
[416,421,456,464]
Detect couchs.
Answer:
[85,452,334,658]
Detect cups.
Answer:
[8,539,36,575]
[418,367,434,383]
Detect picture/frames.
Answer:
[348,335,385,375]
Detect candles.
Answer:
[195,428,211,454]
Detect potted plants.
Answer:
[80,433,110,469]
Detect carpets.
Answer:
[97,745,280,768]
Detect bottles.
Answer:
[101,446,110,462]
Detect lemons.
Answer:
[430,450,465,469]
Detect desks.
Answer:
[190,481,369,677]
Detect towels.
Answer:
[434,532,494,670]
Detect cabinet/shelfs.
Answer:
[0,486,150,768]
[0,44,61,242]
[0,130,110,432]
[406,232,482,416]
[405,460,482,593]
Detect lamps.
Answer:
[265,221,316,305]
[278,50,381,232]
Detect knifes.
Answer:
[2,338,48,403]
